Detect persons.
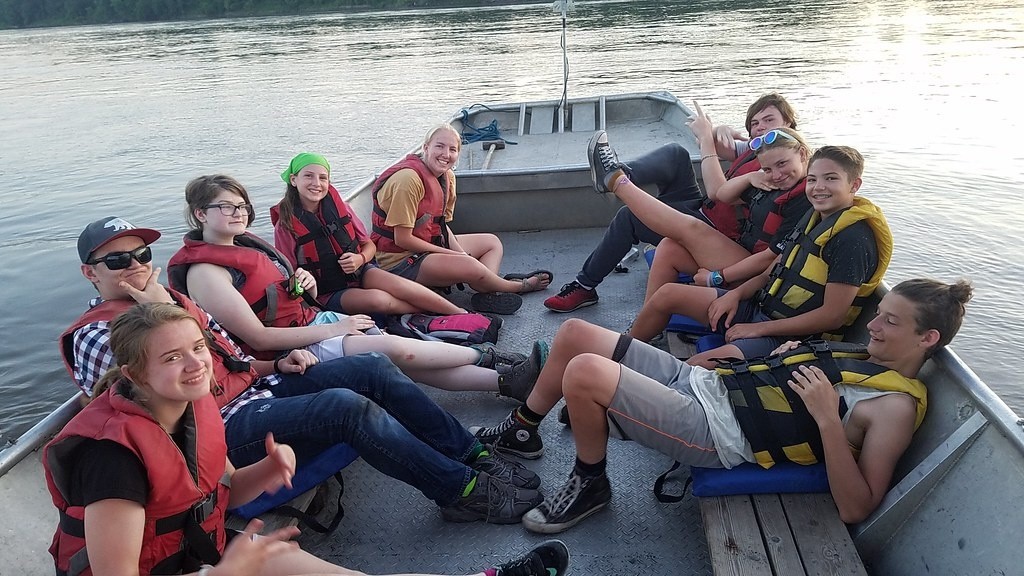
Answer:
[370,123,553,294]
[544,92,797,313]
[626,145,893,370]
[166,175,549,402]
[59,217,544,524]
[270,152,504,331]
[466,279,976,534]
[587,127,814,345]
[42,302,570,576]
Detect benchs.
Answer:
[642,234,868,576]
[221,454,316,545]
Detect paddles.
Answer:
[479,139,506,171]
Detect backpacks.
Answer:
[387,313,498,348]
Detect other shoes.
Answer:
[557,404,571,425]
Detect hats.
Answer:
[78,216,160,263]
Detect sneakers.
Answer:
[587,130,633,194]
[497,340,550,401]
[543,281,598,312]
[440,471,545,524]
[495,539,570,576]
[471,341,527,371]
[521,465,612,534]
[465,442,541,489]
[467,409,544,459]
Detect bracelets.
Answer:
[701,154,718,162]
[360,253,366,267]
[274,354,288,373]
[707,269,729,289]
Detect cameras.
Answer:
[288,274,304,300]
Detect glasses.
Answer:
[87,246,153,270]
[749,129,801,151]
[201,203,252,218]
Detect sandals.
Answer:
[504,269,553,294]
[471,291,523,314]
